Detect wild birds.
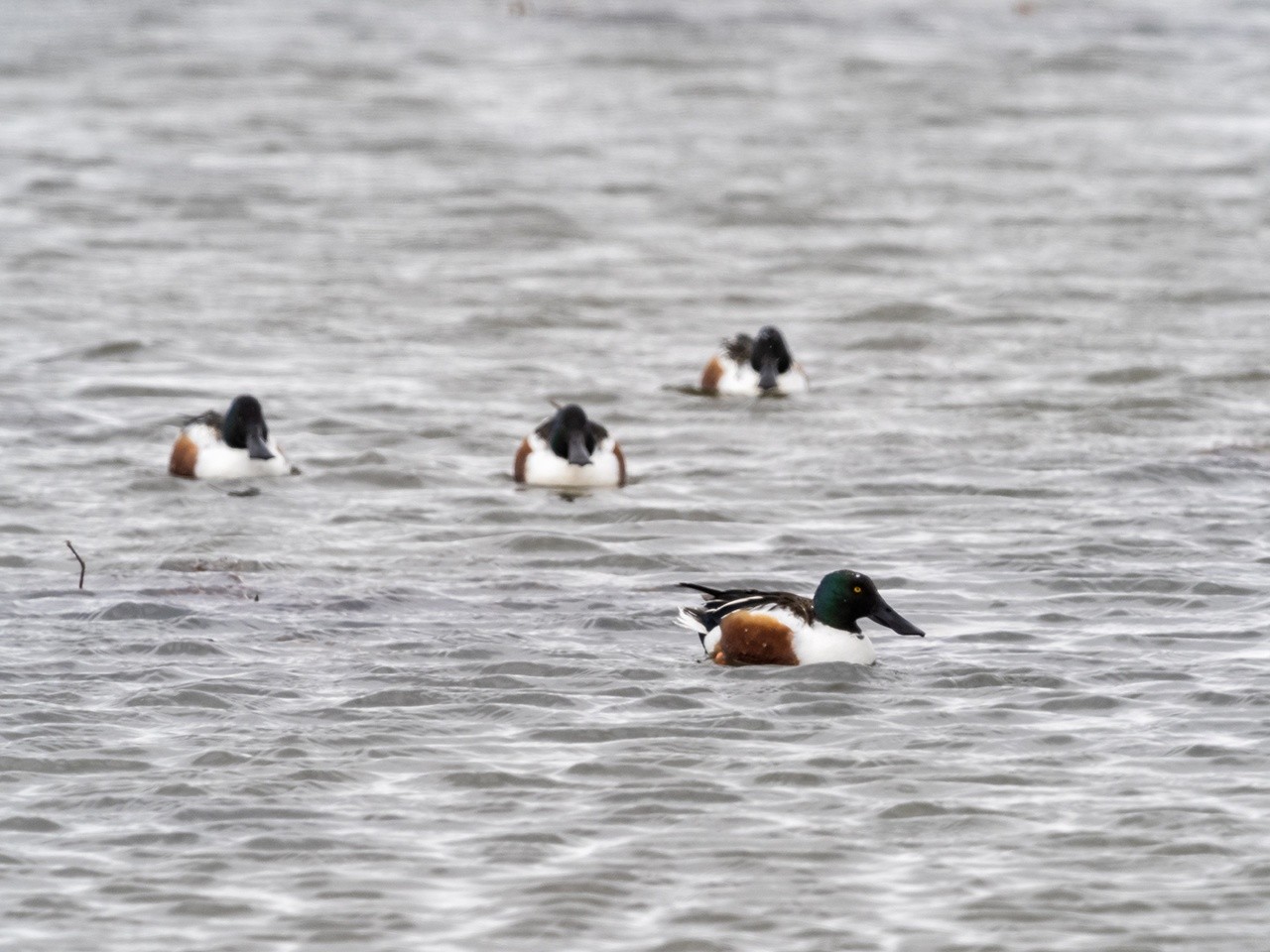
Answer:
[512,405,626,489]
[673,569,927,669]
[166,394,300,478]
[701,326,806,392]
